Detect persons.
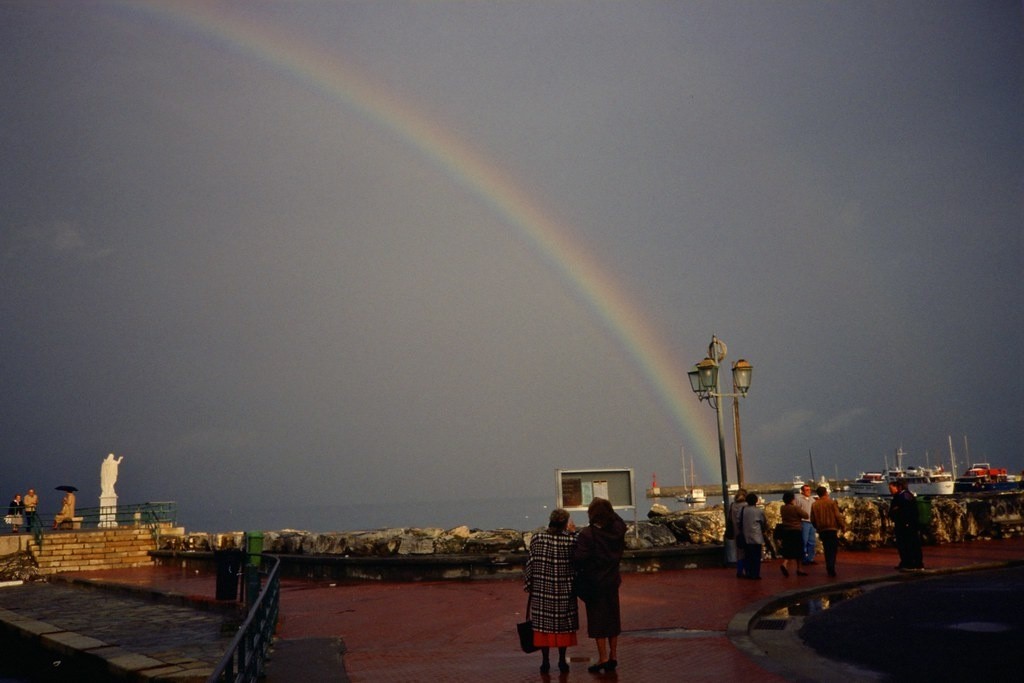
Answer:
[779,491,808,577]
[725,489,770,581]
[24,488,39,532]
[811,486,846,578]
[523,509,579,672]
[796,485,818,565]
[577,498,627,672]
[4,493,25,531]
[52,490,76,528]
[889,478,924,571]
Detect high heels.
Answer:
[588,661,609,672]
[608,659,618,672]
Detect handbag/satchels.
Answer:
[736,532,745,549]
[517,592,542,653]
[571,564,592,603]
[773,524,784,541]
[13,512,23,526]
[724,503,734,540]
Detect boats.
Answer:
[817,476,832,496]
[791,476,805,494]
[674,447,689,502]
[727,484,740,495]
[849,434,954,498]
[686,456,707,503]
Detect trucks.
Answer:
[969,463,1008,479]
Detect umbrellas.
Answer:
[54,486,79,492]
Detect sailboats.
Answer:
[834,465,850,491]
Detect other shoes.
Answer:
[539,663,550,672]
[797,570,807,576]
[803,560,815,564]
[737,574,745,578]
[781,565,789,576]
[752,576,761,580]
[558,662,569,672]
[895,566,906,571]
[907,567,913,572]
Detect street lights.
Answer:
[687,334,754,563]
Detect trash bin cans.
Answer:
[917,494,932,525]
[215,549,244,601]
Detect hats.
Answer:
[550,509,570,522]
